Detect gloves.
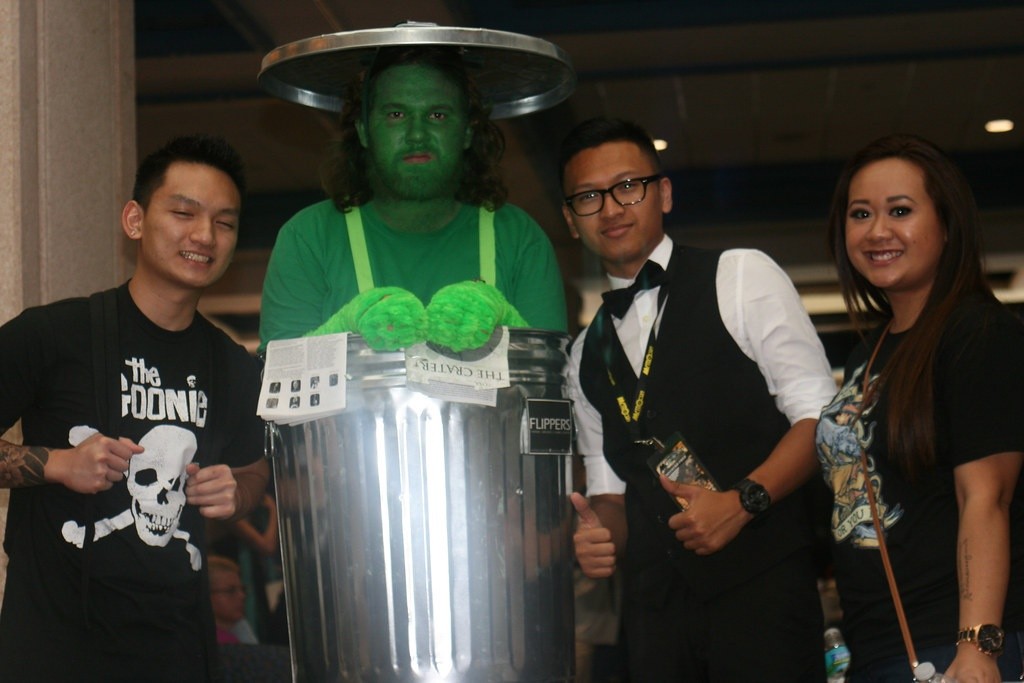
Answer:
[424,280,529,351]
[300,286,430,352]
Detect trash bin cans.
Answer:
[262,330,576,683]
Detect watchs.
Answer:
[730,478,770,512]
[955,624,1006,656]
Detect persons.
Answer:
[557,115,837,683]
[813,133,1023,683]
[0,134,272,683]
[208,556,245,645]
[232,493,277,555]
[257,45,569,354]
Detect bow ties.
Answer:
[601,260,667,320]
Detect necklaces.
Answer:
[858,318,892,415]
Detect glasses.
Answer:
[563,170,669,217]
[208,585,247,600]
